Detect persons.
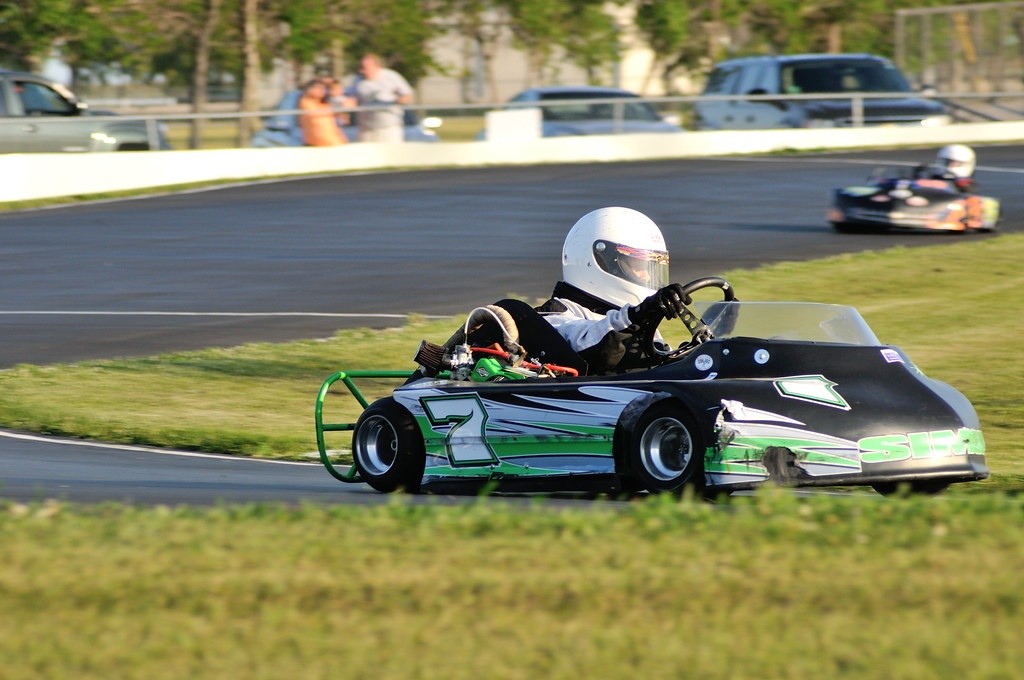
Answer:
[297,80,351,147]
[913,145,976,181]
[355,52,413,143]
[533,207,740,376]
[321,81,356,125]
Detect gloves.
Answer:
[954,177,972,191]
[692,297,740,347]
[645,284,692,321]
[912,163,927,180]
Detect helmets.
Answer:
[562,206,669,310]
[935,144,976,179]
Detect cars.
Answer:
[253,90,439,148]
[694,53,956,130]
[0,71,174,154]
[476,85,681,141]
